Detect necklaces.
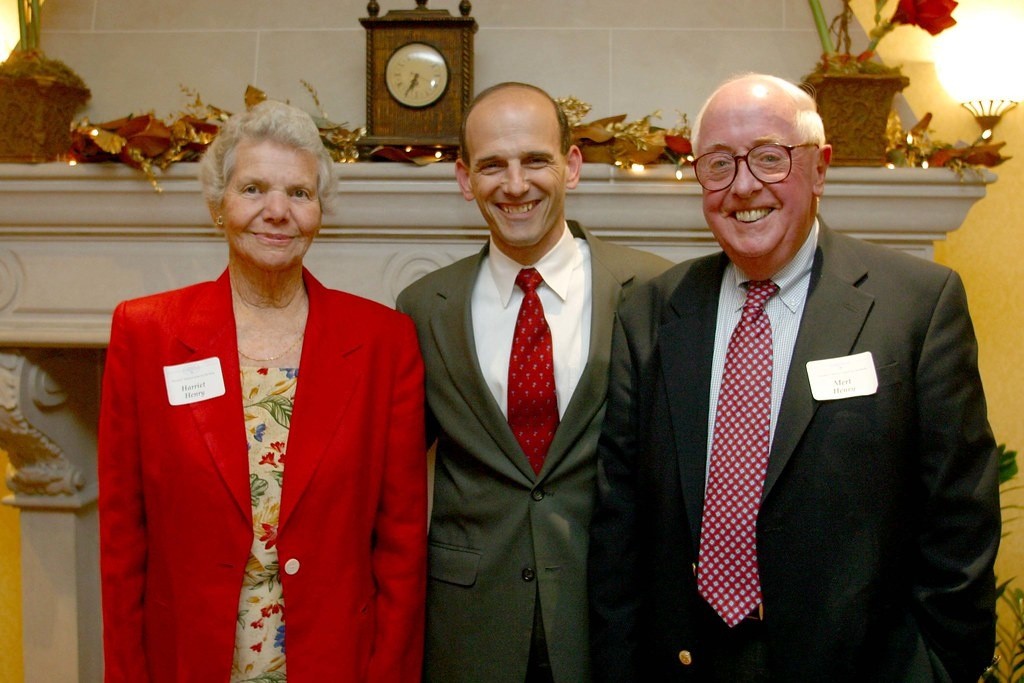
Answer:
[236,335,308,363]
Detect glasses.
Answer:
[689,142,819,192]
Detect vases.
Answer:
[801,72,910,167]
[0,75,91,163]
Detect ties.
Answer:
[507,268,561,481]
[696,277,780,629]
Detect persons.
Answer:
[394,81,681,683]
[582,76,1003,683]
[97,98,431,683]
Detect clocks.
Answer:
[356,0,480,145]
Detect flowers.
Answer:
[806,0,958,73]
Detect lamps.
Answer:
[931,4,1024,143]
